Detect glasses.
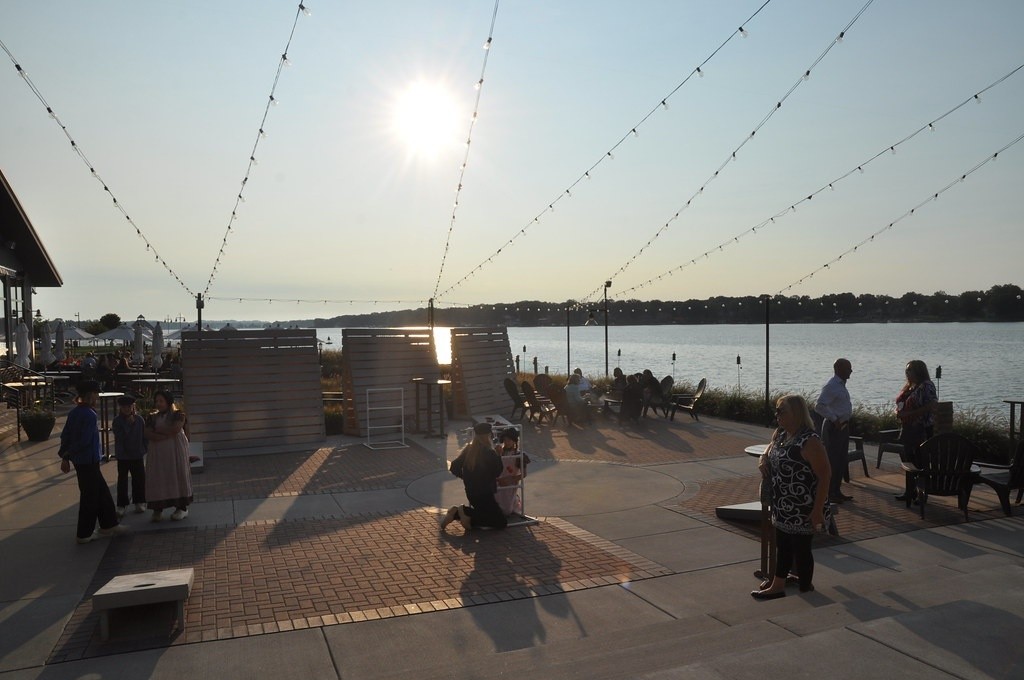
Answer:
[775,407,786,416]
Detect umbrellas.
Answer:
[97,323,299,371]
[55,320,65,360]
[62,326,95,349]
[14,318,31,372]
[40,320,56,369]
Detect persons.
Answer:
[751,395,832,599]
[894,359,939,504]
[439,423,530,530]
[814,358,853,503]
[563,367,663,423]
[111,395,147,517]
[57,378,131,542]
[60,351,181,405]
[142,391,194,521]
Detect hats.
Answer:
[473,423,492,434]
[76,380,107,395]
[117,396,137,405]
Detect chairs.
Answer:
[901,434,975,522]
[504,374,707,426]
[876,401,953,470]
[958,440,1024,517]
[810,408,870,480]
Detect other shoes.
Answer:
[457,506,474,529]
[897,490,917,501]
[752,590,785,599]
[440,507,458,530]
[839,492,854,501]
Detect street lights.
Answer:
[175,317,186,328]
[164,319,172,335]
[75,315,80,328]
[604,280,612,376]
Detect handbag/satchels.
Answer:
[452,444,472,479]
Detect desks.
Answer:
[99,392,125,462]
[744,444,770,458]
[3,371,83,413]
[132,379,180,394]
[118,372,159,379]
[1002,398,1024,464]
[409,377,452,439]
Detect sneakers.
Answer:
[77,531,100,544]
[149,510,161,523]
[99,525,129,535]
[170,506,188,519]
[134,503,144,512]
[115,506,128,515]
